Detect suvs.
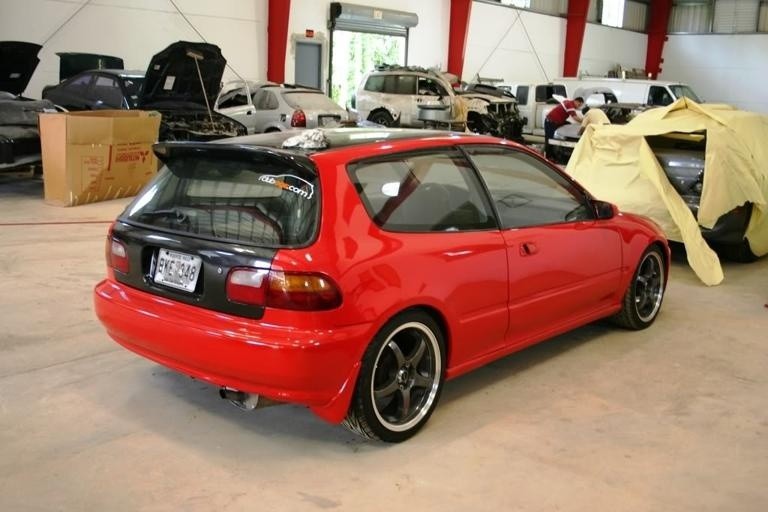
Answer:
[347,68,527,142]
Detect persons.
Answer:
[544,97,584,159]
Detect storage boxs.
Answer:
[38,110,161,207]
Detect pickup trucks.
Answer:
[494,82,617,142]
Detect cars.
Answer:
[43,42,248,179]
[214,80,359,136]
[547,102,663,163]
[95,127,670,443]
[567,111,767,265]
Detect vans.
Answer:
[554,77,704,105]
